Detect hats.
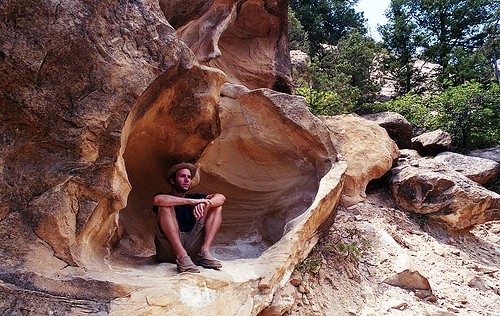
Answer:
[166,161,197,185]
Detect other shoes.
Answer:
[194,251,222,268]
[176,256,200,273]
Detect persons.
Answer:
[152,163,227,273]
